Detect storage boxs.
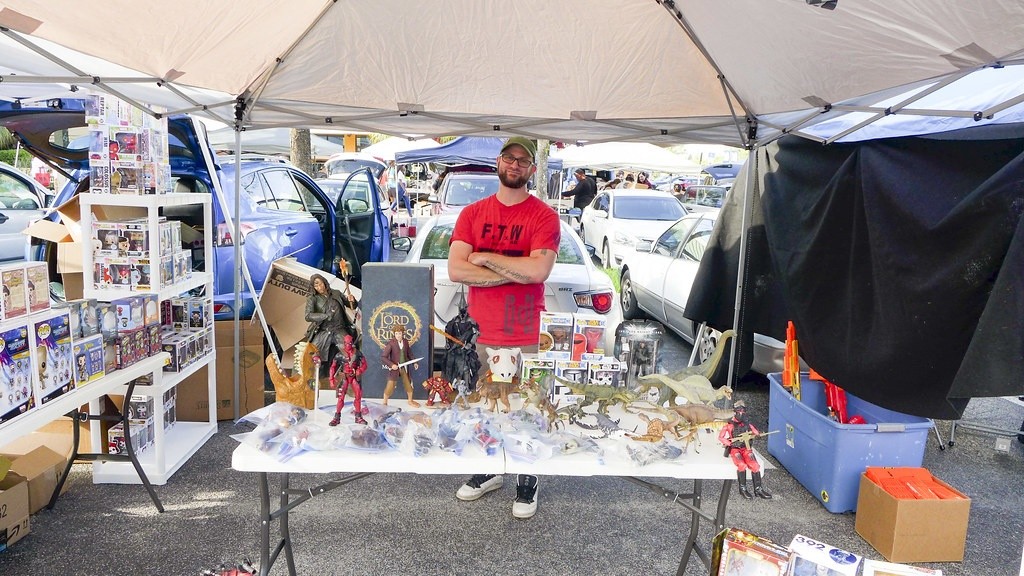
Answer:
[23,392,124,455]
[853,472,971,564]
[0,481,30,552]
[765,370,933,514]
[0,446,70,514]
[20,99,212,388]
[173,318,264,423]
[246,238,361,350]
[517,309,621,407]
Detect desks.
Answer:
[232,398,767,576]
[0,351,174,515]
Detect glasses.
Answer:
[500,154,534,168]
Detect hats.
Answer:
[500,136,536,163]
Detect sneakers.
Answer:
[513,474,538,519]
[456,474,503,500]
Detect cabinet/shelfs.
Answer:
[77,190,218,486]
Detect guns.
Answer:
[723,430,780,458]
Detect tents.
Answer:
[203,124,742,205]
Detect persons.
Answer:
[719,399,773,499]
[381,160,686,218]
[32,155,45,183]
[382,324,421,408]
[448,136,562,515]
[305,274,359,398]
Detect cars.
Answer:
[620,212,810,389]
[0,161,54,265]
[656,175,705,191]
[679,163,744,212]
[423,172,499,215]
[311,178,393,241]
[579,189,692,270]
[392,215,624,363]
[0,98,390,322]
